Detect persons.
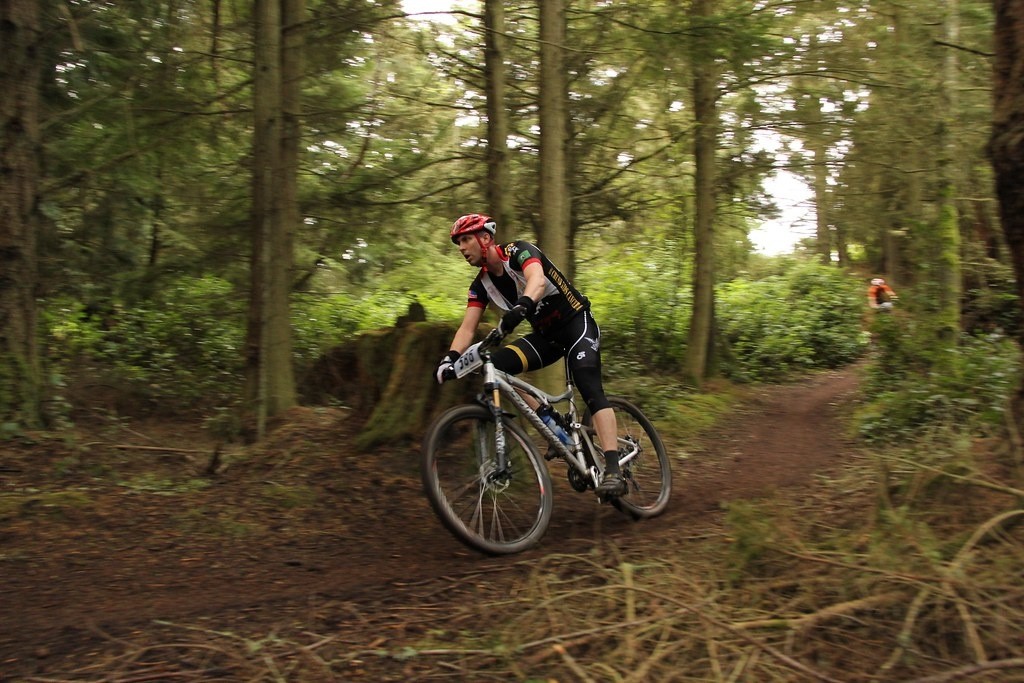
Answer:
[869,279,899,348]
[436,214,630,497]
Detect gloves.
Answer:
[436,355,455,383]
[499,308,518,337]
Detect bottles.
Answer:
[540,415,576,451]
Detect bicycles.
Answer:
[420,329,672,556]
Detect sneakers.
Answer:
[544,446,560,460]
[595,468,629,495]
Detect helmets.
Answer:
[450,214,496,239]
